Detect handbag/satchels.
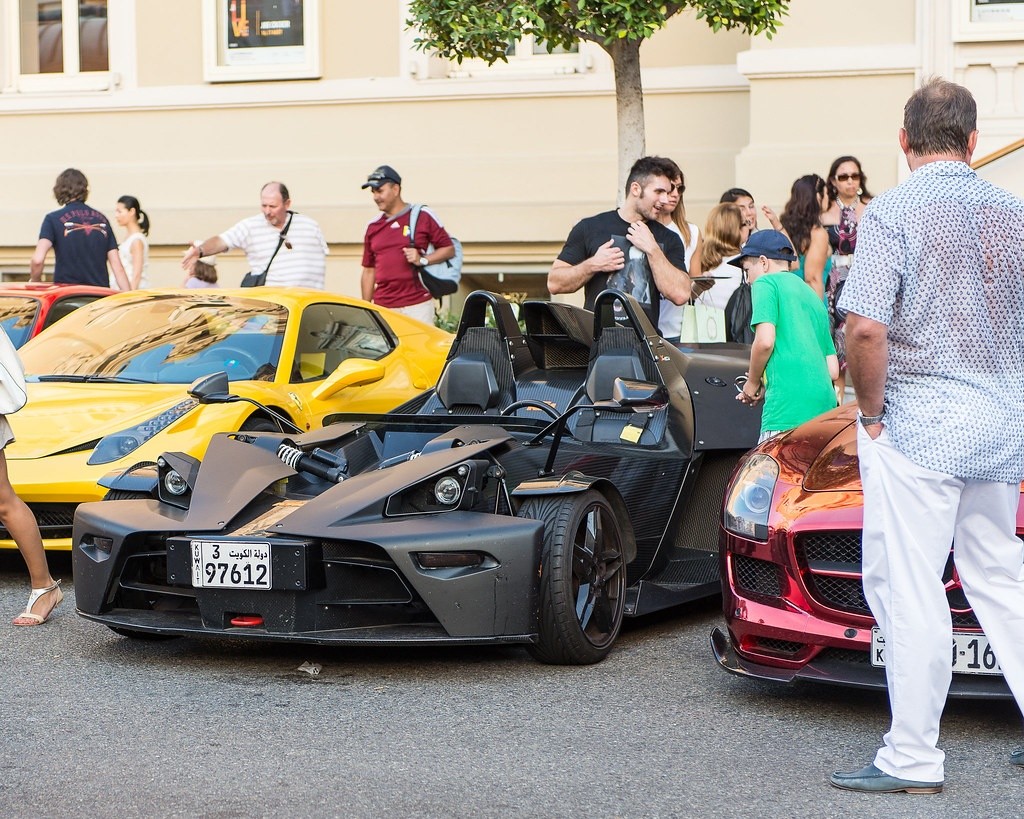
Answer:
[240,272,267,287]
[725,270,755,345]
[409,202,463,300]
[680,280,726,344]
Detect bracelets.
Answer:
[198,246,202,258]
[857,409,885,425]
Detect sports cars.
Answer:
[70,287,765,668]
[0,285,455,553]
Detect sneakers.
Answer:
[829,762,944,794]
[1009,750,1024,767]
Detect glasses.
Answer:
[738,221,747,230]
[836,173,863,182]
[669,182,685,194]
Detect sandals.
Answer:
[13,577,64,626]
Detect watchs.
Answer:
[420,255,428,266]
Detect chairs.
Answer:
[414,326,519,417]
[575,327,668,446]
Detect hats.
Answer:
[183,241,216,266]
[361,165,401,189]
[726,229,798,269]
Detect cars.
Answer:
[0,282,119,353]
[712,404,1024,700]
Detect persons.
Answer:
[735,229,839,446]
[31,168,132,291]
[0,323,65,626]
[546,155,873,405]
[108,195,150,291]
[180,181,329,290]
[183,240,221,289]
[828,75,1024,794]
[361,165,455,328]
